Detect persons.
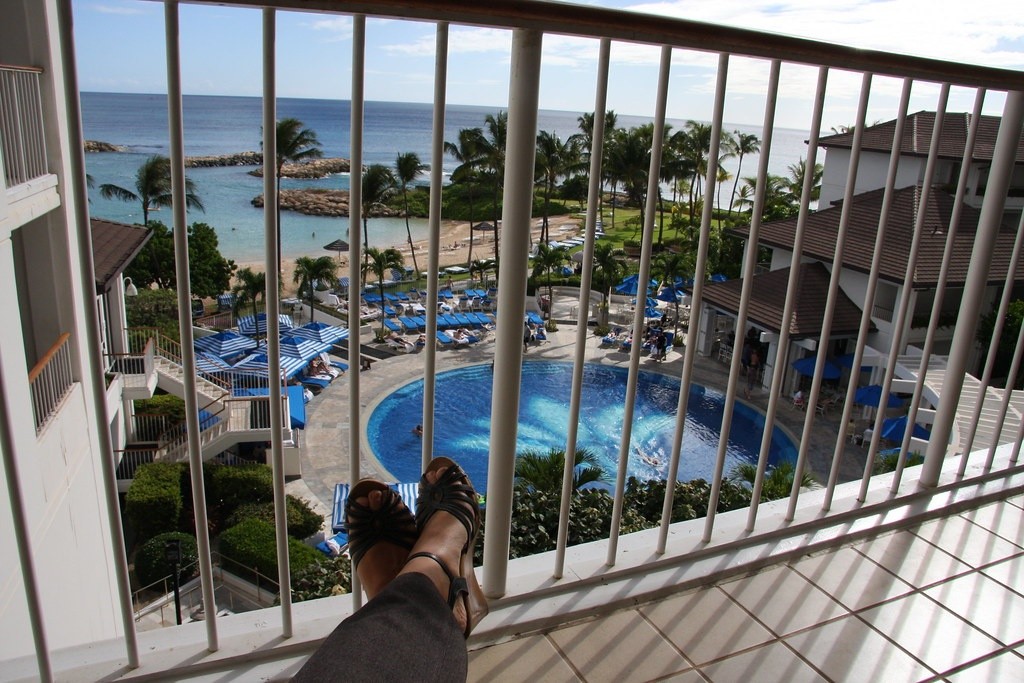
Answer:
[606,328,667,362]
[845,419,856,428]
[729,325,768,399]
[454,328,479,341]
[384,332,414,347]
[419,333,426,342]
[862,425,874,435]
[292,457,490,683]
[309,358,330,374]
[412,424,423,436]
[793,386,807,410]
[523,321,531,352]
[454,241,458,248]
[444,276,454,290]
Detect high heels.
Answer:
[345,477,417,571]
[403,457,489,640]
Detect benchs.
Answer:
[624,245,640,257]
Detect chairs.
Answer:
[717,342,732,364]
[362,287,546,353]
[602,326,674,360]
[191,299,204,317]
[793,384,873,448]
[294,361,348,396]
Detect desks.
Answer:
[678,304,690,320]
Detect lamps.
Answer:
[123,277,138,298]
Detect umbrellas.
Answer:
[615,274,727,320]
[791,349,930,464]
[193,312,349,431]
[324,239,349,262]
[332,482,420,531]
[472,222,494,239]
